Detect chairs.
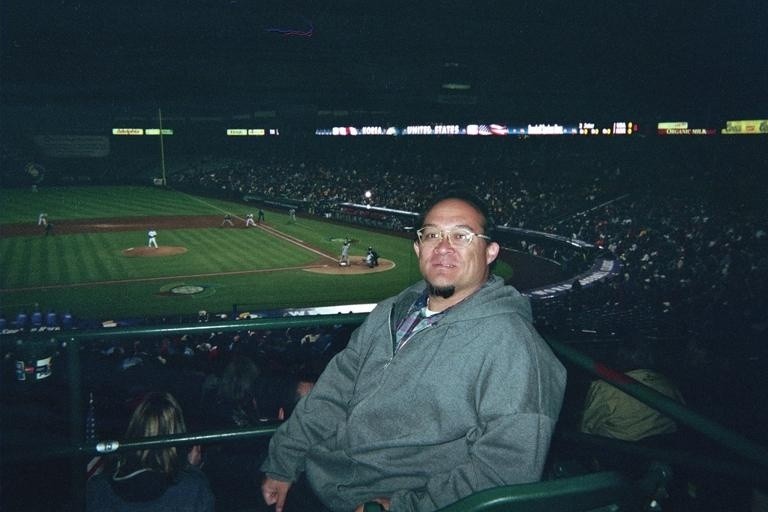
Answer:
[0,291,690,512]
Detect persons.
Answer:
[2,278,360,512]
[259,188,568,512]
[37,150,765,277]
[532,277,767,511]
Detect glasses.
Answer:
[417,226,490,249]
